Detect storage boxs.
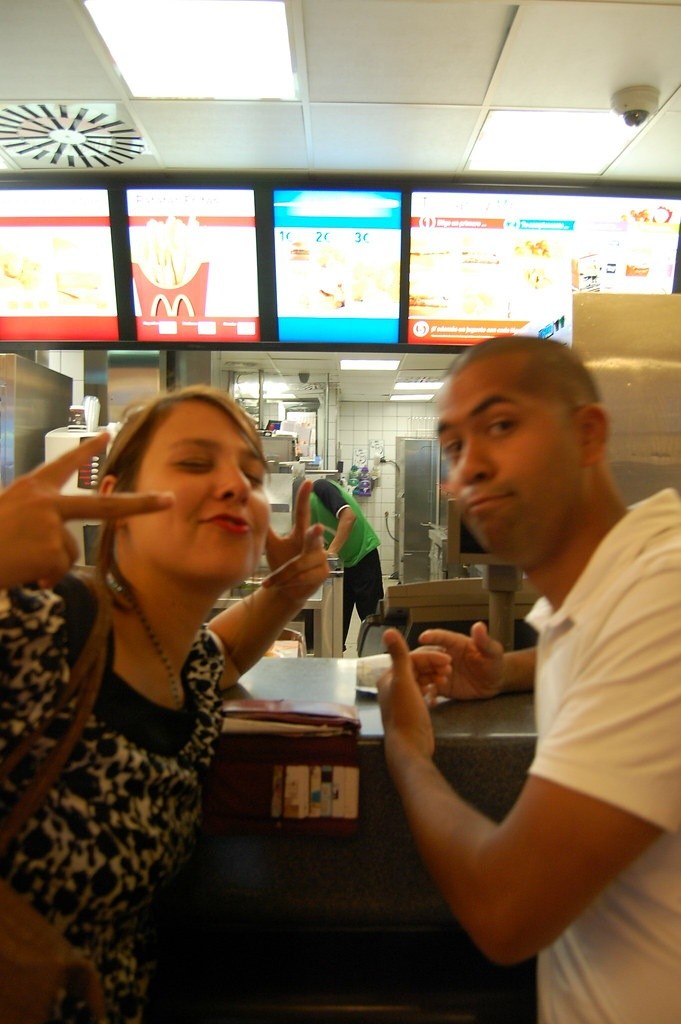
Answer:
[263,627,305,659]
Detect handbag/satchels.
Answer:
[0,879,107,1024]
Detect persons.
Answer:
[293,476,384,653]
[375,336,681,1024]
[0,385,330,1024]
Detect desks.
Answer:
[212,552,344,660]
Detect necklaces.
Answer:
[106,574,183,712]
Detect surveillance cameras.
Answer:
[611,86,660,128]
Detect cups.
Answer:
[67,405,87,433]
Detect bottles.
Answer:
[348,466,372,497]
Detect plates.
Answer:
[345,652,392,693]
